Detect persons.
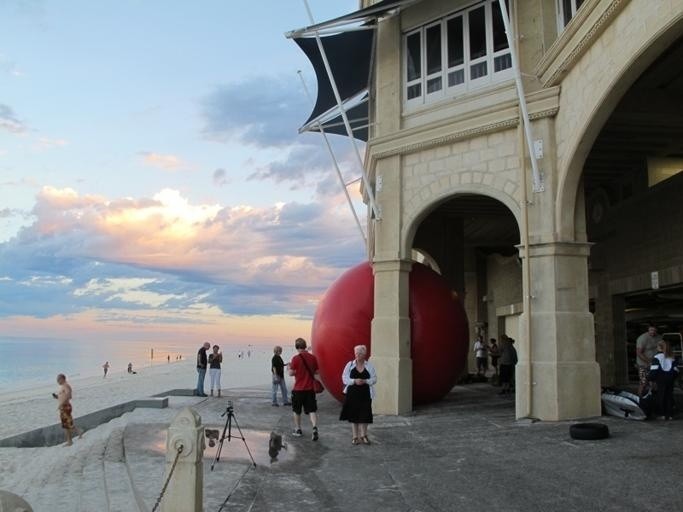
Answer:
[269,345,292,407]
[100,361,110,378]
[285,337,321,442]
[471,334,489,376]
[485,337,499,376]
[206,344,222,398]
[50,372,83,448]
[645,337,680,421]
[191,341,210,398]
[126,362,134,374]
[494,337,516,394]
[486,334,508,356]
[336,343,378,446]
[633,322,663,398]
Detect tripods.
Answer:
[210,414,257,471]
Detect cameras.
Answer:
[225,399,235,413]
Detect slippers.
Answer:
[359,435,369,444]
[351,436,358,445]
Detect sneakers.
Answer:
[292,428,302,437]
[283,402,291,406]
[272,404,279,407]
[654,415,666,421]
[200,393,208,397]
[668,415,673,421]
[312,428,319,441]
[496,389,510,395]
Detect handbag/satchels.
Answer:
[312,379,324,393]
